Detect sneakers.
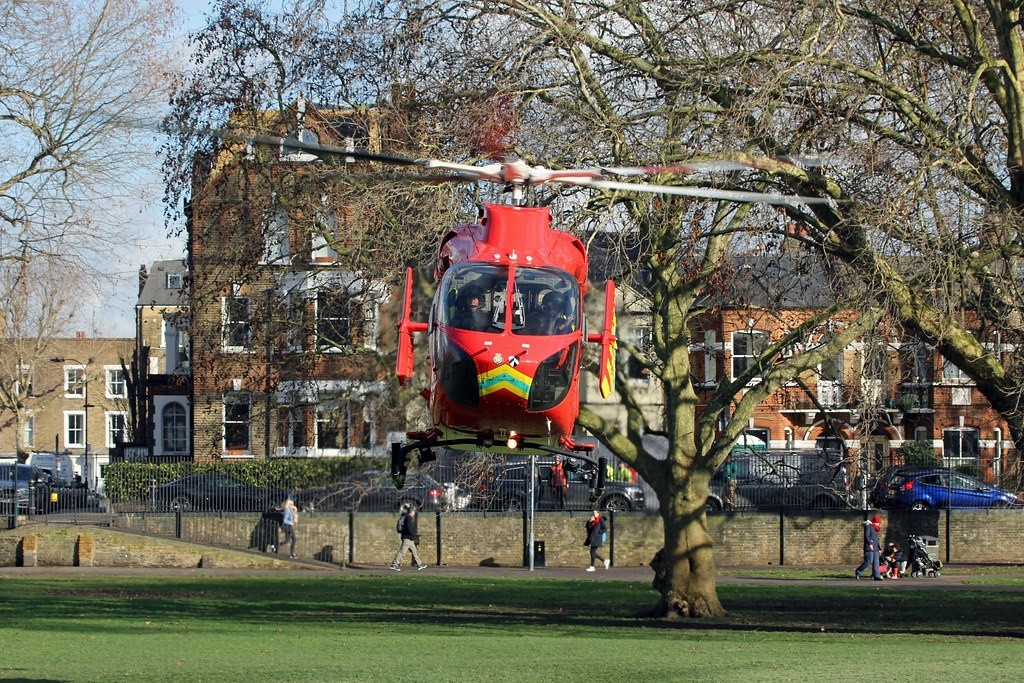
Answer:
[855,571,860,580]
[271,544,277,552]
[604,558,611,570]
[418,563,427,570]
[585,566,595,572]
[390,563,400,571]
[874,576,883,580]
[290,554,297,559]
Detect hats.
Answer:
[408,507,418,513]
[554,456,562,461]
[401,503,411,513]
[871,516,881,523]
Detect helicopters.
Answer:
[252,135,828,501]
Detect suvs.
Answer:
[468,460,647,513]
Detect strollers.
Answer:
[907,535,944,578]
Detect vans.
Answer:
[705,454,851,510]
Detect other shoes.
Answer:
[892,575,897,579]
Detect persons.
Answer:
[879,539,908,579]
[525,291,569,335]
[455,283,490,329]
[854,512,883,580]
[583,509,610,573]
[549,454,569,511]
[276,498,299,559]
[602,457,640,484]
[389,500,429,572]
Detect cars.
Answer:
[0,462,52,514]
[150,473,287,513]
[872,466,1021,509]
[291,471,450,513]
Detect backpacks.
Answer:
[396,515,405,532]
[602,533,606,543]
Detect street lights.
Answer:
[50,357,88,507]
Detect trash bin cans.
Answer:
[921,536,941,576]
[534,541,546,567]
